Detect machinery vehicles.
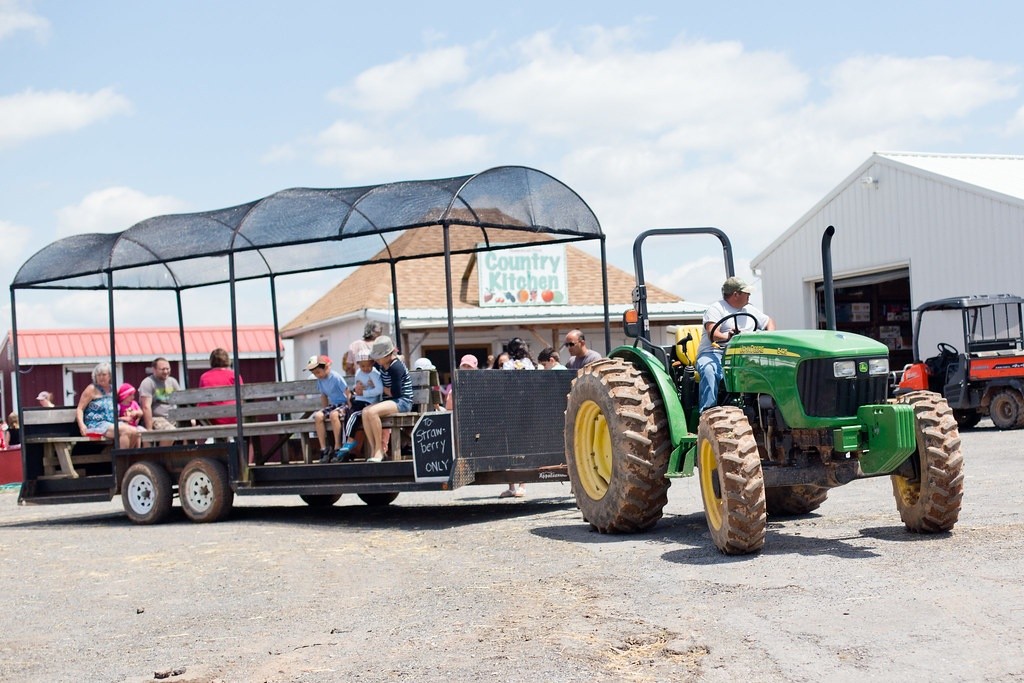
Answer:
[891,293,1024,432]
[9,165,965,554]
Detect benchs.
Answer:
[23,408,115,480]
[139,369,441,466]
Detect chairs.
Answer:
[948,353,967,385]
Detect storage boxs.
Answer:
[875,326,903,350]
[849,302,870,322]
[882,303,909,321]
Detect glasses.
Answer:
[565,340,580,347]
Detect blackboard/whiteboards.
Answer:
[410,412,455,483]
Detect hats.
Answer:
[36,391,49,400]
[460,354,477,369]
[415,358,437,370]
[355,350,374,364]
[508,337,528,358]
[303,355,327,370]
[117,383,135,402]
[370,335,394,359]
[722,277,754,295]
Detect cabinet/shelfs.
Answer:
[818,297,912,330]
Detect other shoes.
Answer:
[367,452,385,462]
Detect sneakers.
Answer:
[340,440,358,451]
[331,449,340,463]
[337,451,346,462]
[319,446,333,463]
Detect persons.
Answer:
[76,362,151,449]
[197,349,244,443]
[36,391,54,407]
[138,357,196,446]
[414,358,442,410]
[336,319,414,462]
[486,337,545,497]
[303,355,351,462]
[695,276,774,415]
[445,355,478,410]
[537,347,566,369]
[564,329,602,368]
[0,413,21,447]
